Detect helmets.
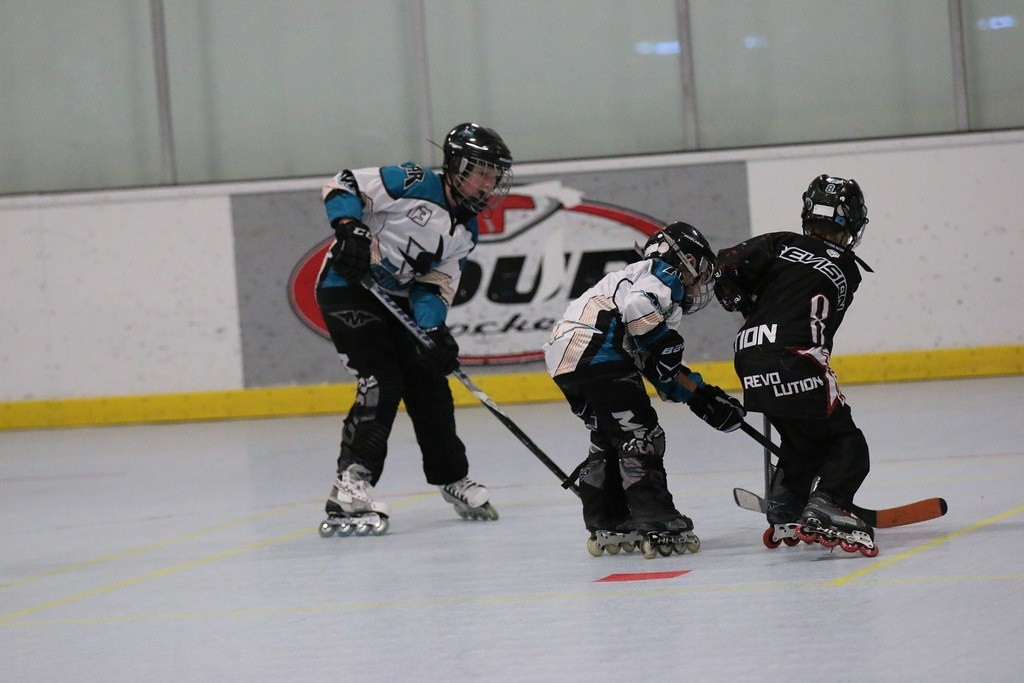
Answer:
[443,122,513,217]
[643,220,716,315]
[801,174,868,238]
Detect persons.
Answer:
[713,175,878,560]
[315,122,513,537]
[543,221,747,558]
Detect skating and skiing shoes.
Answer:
[440,476,499,521]
[319,463,389,537]
[795,475,879,557]
[635,514,700,559]
[763,468,801,548]
[587,519,642,557]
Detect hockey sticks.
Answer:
[662,366,948,528]
[358,271,582,498]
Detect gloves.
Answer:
[642,329,684,393]
[331,223,372,285]
[688,384,746,432]
[416,323,459,374]
